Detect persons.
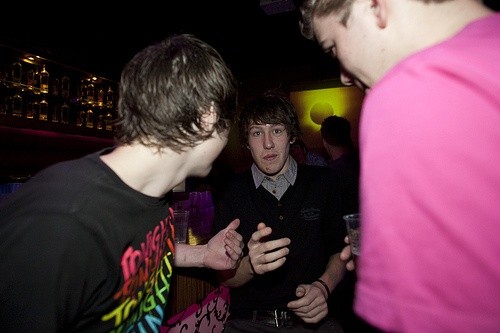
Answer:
[302,0,500,333]
[0,33,245,333]
[216,90,367,333]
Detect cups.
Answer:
[173,211,188,244]
[343,213,360,256]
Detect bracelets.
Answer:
[316,278,331,300]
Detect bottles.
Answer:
[51,99,69,125]
[98,83,119,112]
[0,89,24,117]
[25,91,49,121]
[1,60,28,90]
[51,65,71,97]
[28,62,50,94]
[96,110,114,132]
[80,78,97,102]
[74,105,94,129]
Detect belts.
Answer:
[235,309,299,328]
